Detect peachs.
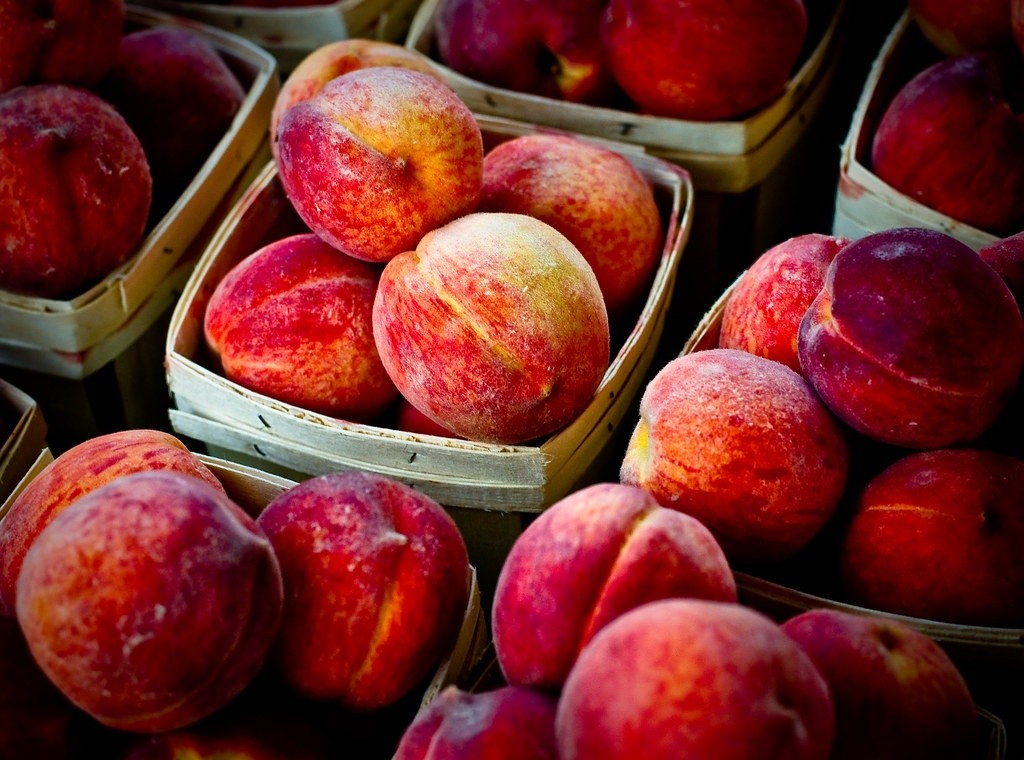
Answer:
[0,0,1024,760]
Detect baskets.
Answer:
[1,0,1024,760]
